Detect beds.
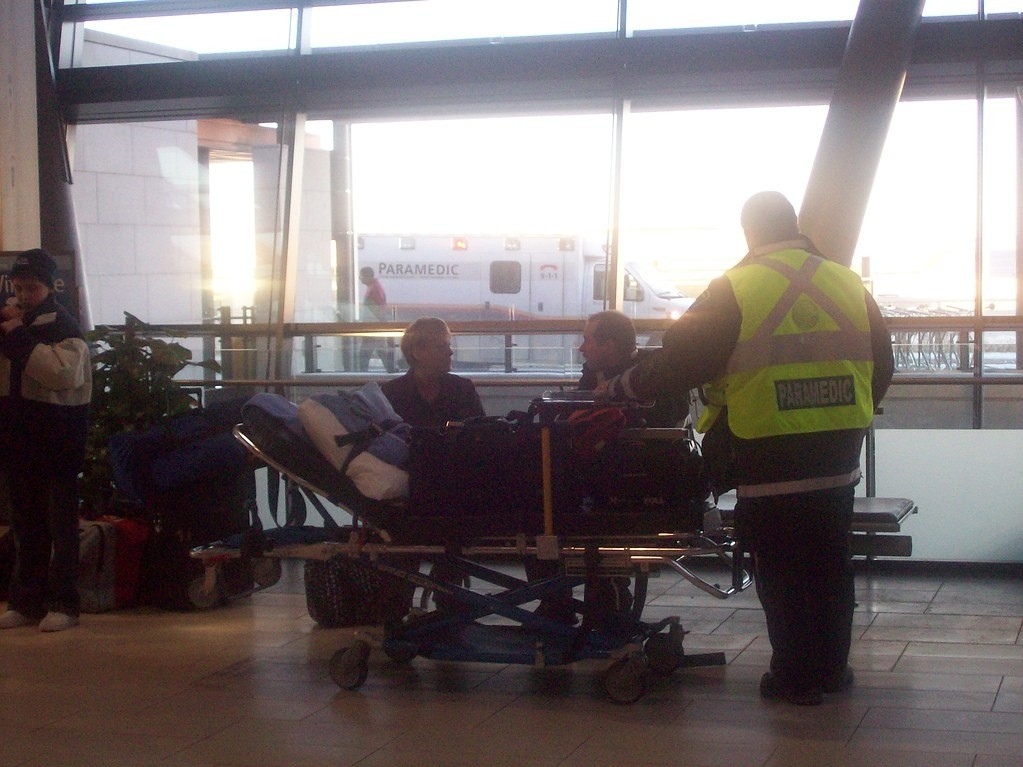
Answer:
[188,392,922,707]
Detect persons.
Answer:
[594,190,895,704]
[1,249,92,632]
[359,267,395,374]
[382,317,486,503]
[522,311,656,634]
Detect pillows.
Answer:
[296,379,414,500]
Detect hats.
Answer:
[13,248,57,288]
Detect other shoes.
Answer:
[822,662,854,690]
[762,671,821,705]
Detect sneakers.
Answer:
[39,612,79,632]
[0,610,35,628]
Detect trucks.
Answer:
[333,232,693,371]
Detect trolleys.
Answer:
[187,397,756,705]
